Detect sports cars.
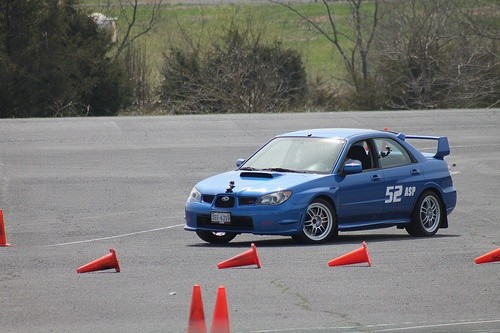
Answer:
[183,127,457,246]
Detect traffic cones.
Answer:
[0,209,10,246]
[217,243,264,269]
[77,248,121,274]
[475,247,500,264]
[187,285,231,333]
[327,241,372,268]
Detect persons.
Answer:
[327,146,352,173]
